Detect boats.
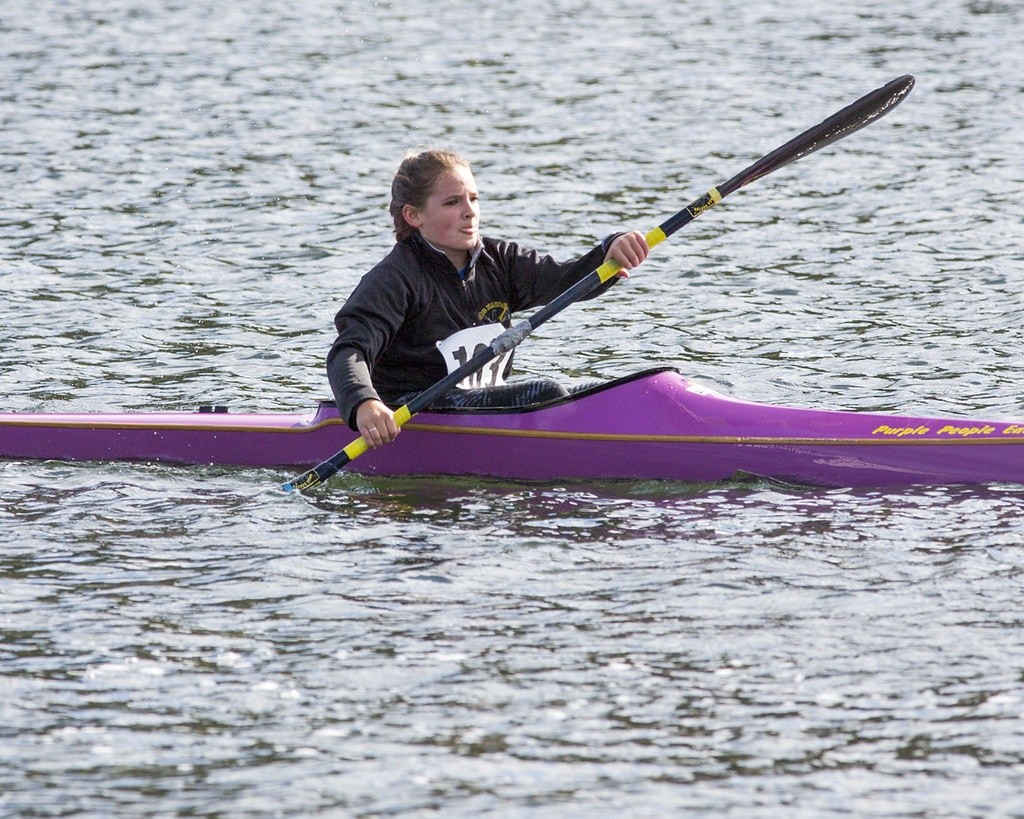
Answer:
[0,365,1024,490]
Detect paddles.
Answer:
[281,74,917,492]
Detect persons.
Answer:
[326,151,650,449]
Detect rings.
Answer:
[369,428,376,433]
